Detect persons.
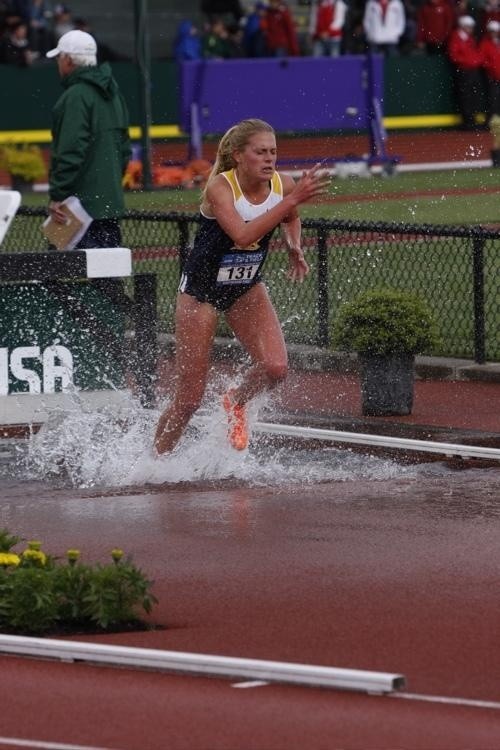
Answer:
[45,29,134,251]
[0,0,90,67]
[152,119,333,461]
[176,0,405,64]
[415,1,500,131]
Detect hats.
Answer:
[45,30,97,58]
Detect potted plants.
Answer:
[0,139,46,192]
[329,287,448,417]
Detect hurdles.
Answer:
[0,246,158,430]
[178,52,404,176]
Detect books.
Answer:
[42,203,84,250]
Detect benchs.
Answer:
[40,1,310,58]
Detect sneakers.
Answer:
[222,391,249,450]
